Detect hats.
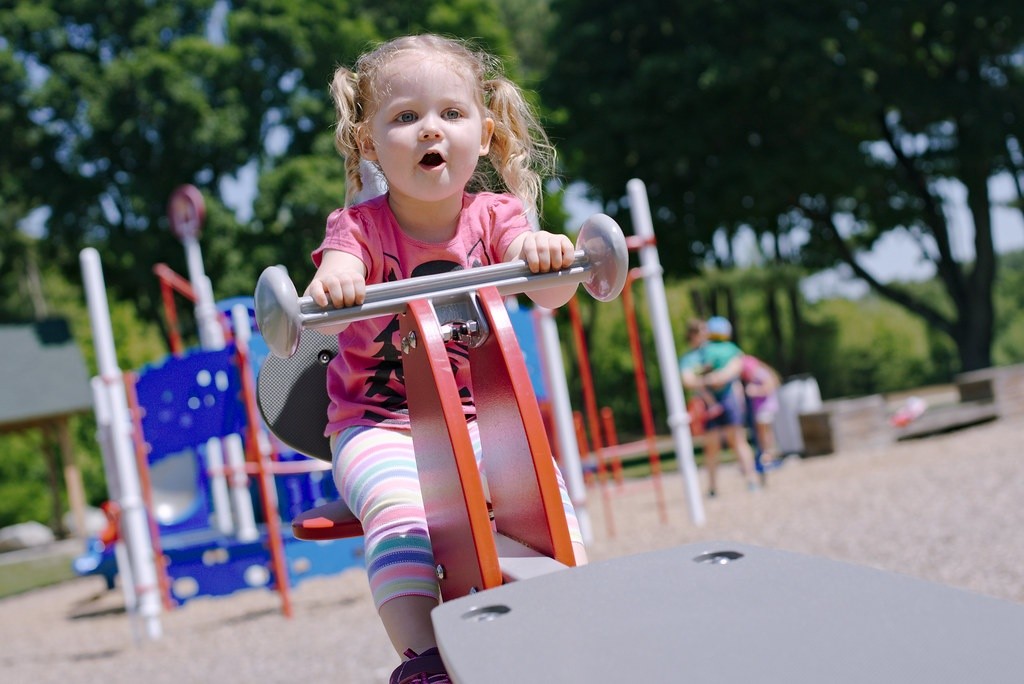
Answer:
[707,318,731,335]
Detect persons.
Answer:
[301,34,579,684]
[679,315,779,498]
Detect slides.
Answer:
[145,449,213,532]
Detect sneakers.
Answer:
[389,647,453,684]
